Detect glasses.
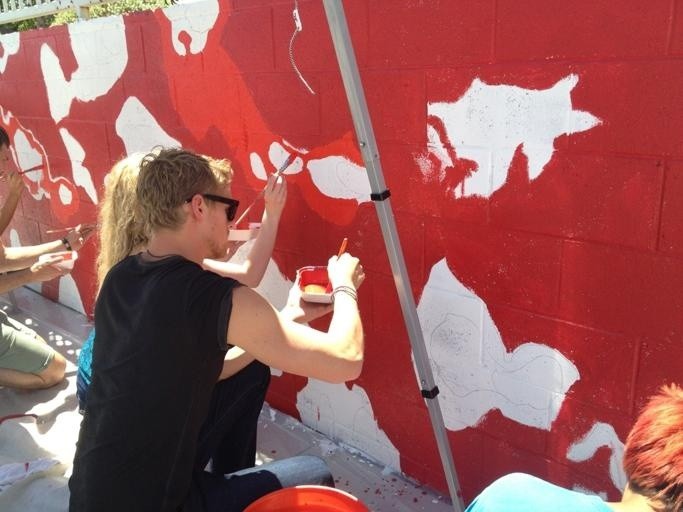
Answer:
[187,190,241,221]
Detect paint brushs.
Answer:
[325,238,348,294]
[46,224,97,234]
[234,152,296,225]
[0,165,44,180]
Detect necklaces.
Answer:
[144,248,176,260]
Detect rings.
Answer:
[78,236,83,243]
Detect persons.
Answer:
[75,152,287,413]
[461,381,683,511]
[0,128,96,390]
[66,144,367,509]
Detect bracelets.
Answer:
[59,236,72,253]
[329,286,358,302]
